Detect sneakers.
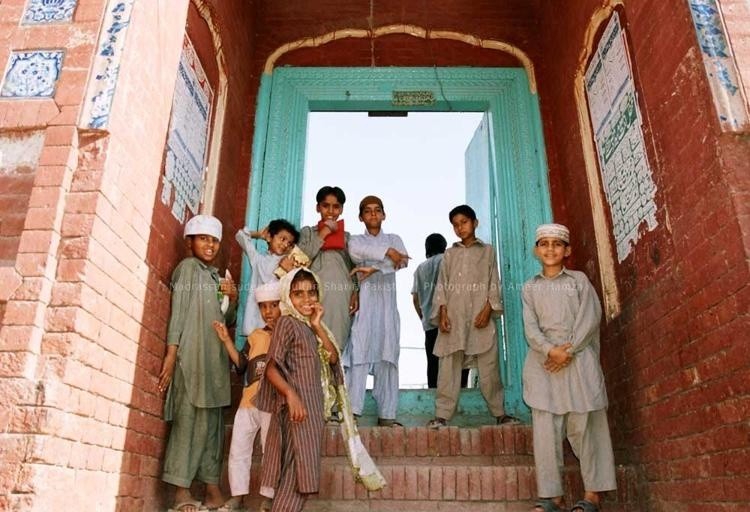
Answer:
[377,418,403,427]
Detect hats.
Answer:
[535,223,570,243]
[255,282,280,303]
[183,214,223,243]
[359,195,384,209]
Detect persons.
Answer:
[254,268,386,512]
[410,234,470,388]
[297,185,359,357]
[212,279,283,512]
[159,214,238,512]
[427,204,522,429]
[347,195,409,430]
[520,223,618,512]
[235,218,300,350]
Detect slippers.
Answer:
[217,498,248,512]
[260,501,272,512]
[534,499,561,512]
[569,500,598,512]
[167,501,209,512]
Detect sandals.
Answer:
[426,417,447,428]
[497,414,521,425]
